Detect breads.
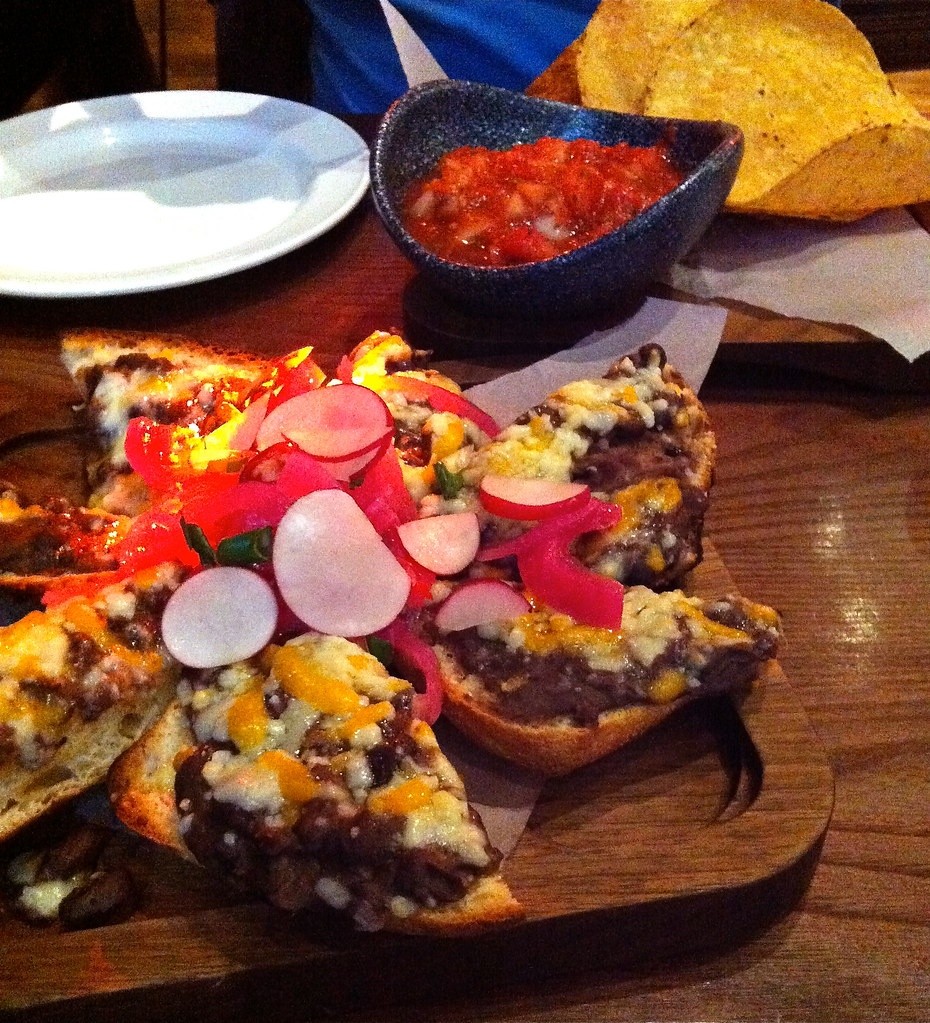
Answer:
[50,325,277,514]
[437,341,713,577]
[0,562,187,842]
[412,578,780,773]
[321,366,464,500]
[0,493,131,591]
[108,633,529,938]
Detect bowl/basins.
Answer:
[371,79,744,307]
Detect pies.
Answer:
[576,0,930,222]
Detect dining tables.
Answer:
[1,1,930,1023]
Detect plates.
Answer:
[0,89,369,299]
[0,405,835,1022]
